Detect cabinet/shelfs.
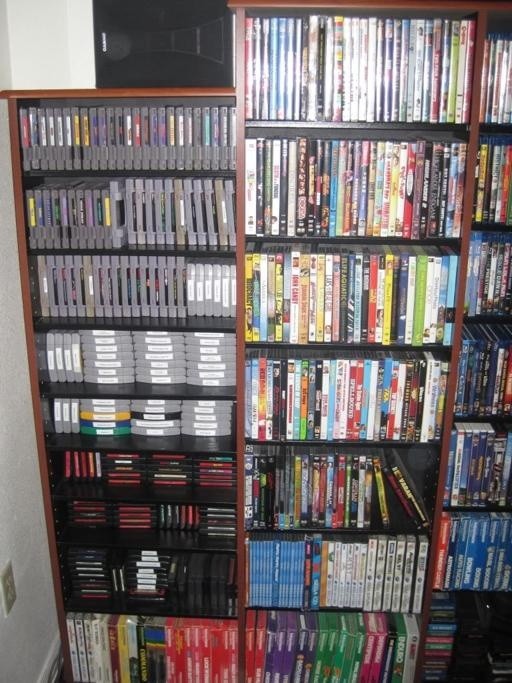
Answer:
[1,1,511,683]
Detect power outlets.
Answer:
[0,559,17,617]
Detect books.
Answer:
[479,35,511,123]
[242,13,477,126]
[484,637,511,681]
[456,326,510,416]
[16,106,235,172]
[31,250,239,330]
[247,535,429,616]
[246,608,420,680]
[20,172,237,256]
[423,596,456,682]
[59,449,237,504]
[242,445,431,535]
[435,517,512,595]
[473,142,510,227]
[63,609,236,681]
[70,548,237,616]
[243,136,466,240]
[66,498,235,541]
[246,244,458,348]
[45,328,234,399]
[45,392,233,451]
[461,227,511,323]
[442,419,511,514]
